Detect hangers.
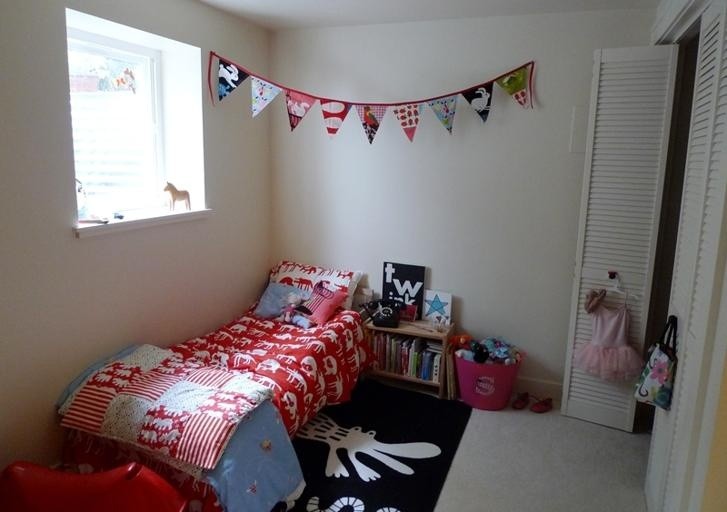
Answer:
[588,274,639,301]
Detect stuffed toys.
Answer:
[272,291,303,324]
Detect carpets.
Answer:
[270,381,472,512]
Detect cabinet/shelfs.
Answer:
[363,318,456,400]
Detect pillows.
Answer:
[254,259,363,330]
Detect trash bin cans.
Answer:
[455,356,518,410]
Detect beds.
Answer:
[71,291,377,510]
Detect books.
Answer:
[371,330,443,384]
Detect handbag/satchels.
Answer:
[633,315,679,413]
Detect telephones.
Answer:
[369,299,406,328]
